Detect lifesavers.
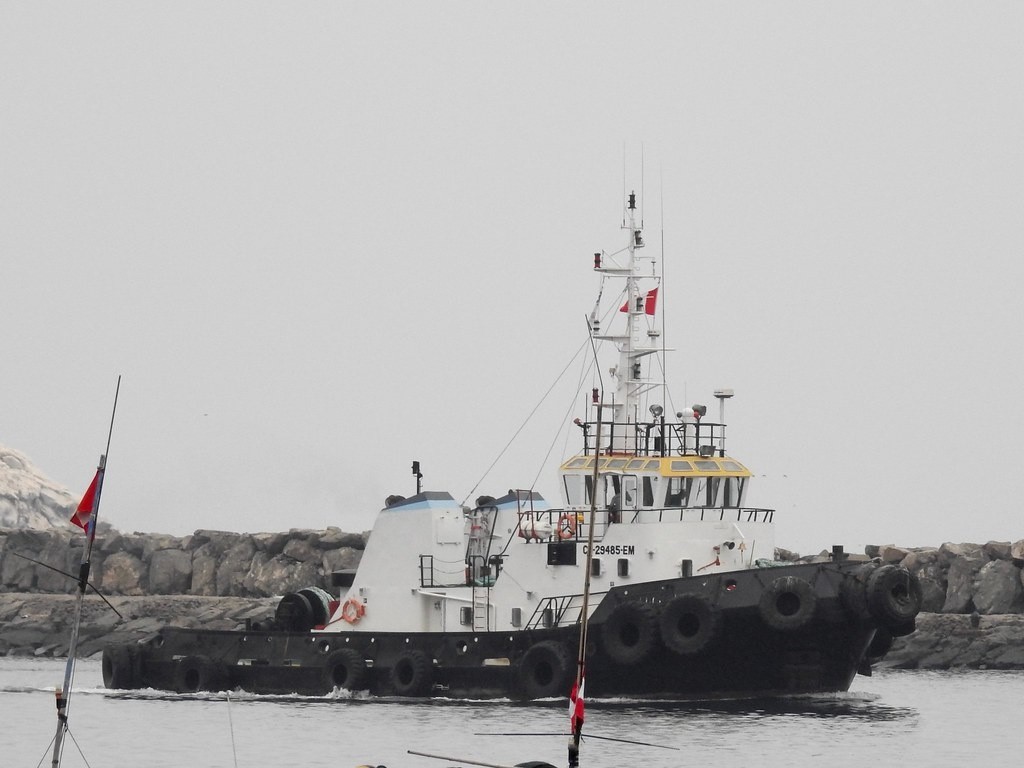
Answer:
[175,655,218,696]
[601,600,662,667]
[658,592,725,659]
[558,515,576,538]
[865,563,924,628]
[101,642,133,691]
[758,576,820,633]
[323,648,368,695]
[867,628,892,659]
[343,599,362,624]
[387,650,436,697]
[519,640,577,698]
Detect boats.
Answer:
[96,141,925,701]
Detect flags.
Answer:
[569,673,585,737]
[620,286,658,315]
[69,467,101,542]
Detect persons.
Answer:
[668,489,686,506]
[609,488,620,523]
